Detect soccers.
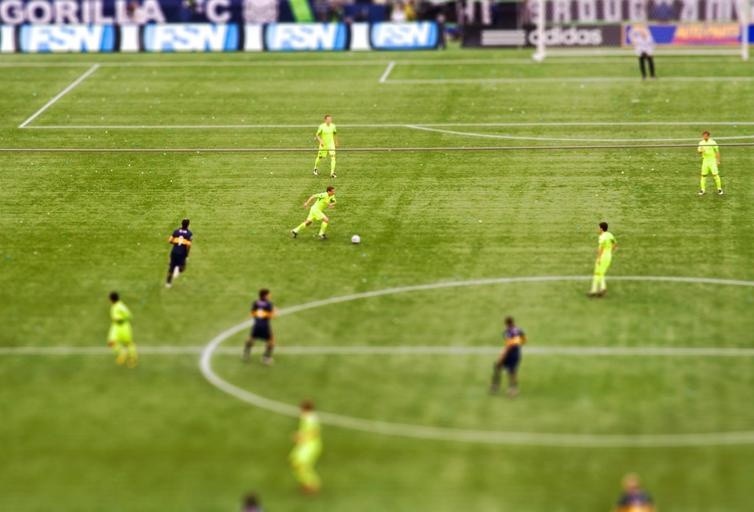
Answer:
[352,235,360,243]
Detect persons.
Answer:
[166,218,194,287]
[616,473,655,512]
[240,287,279,366]
[696,131,724,194]
[313,113,340,181]
[588,222,617,294]
[287,400,326,494]
[489,317,528,401]
[292,186,337,241]
[633,28,658,80]
[240,494,264,511]
[106,292,138,372]
[170,1,440,22]
